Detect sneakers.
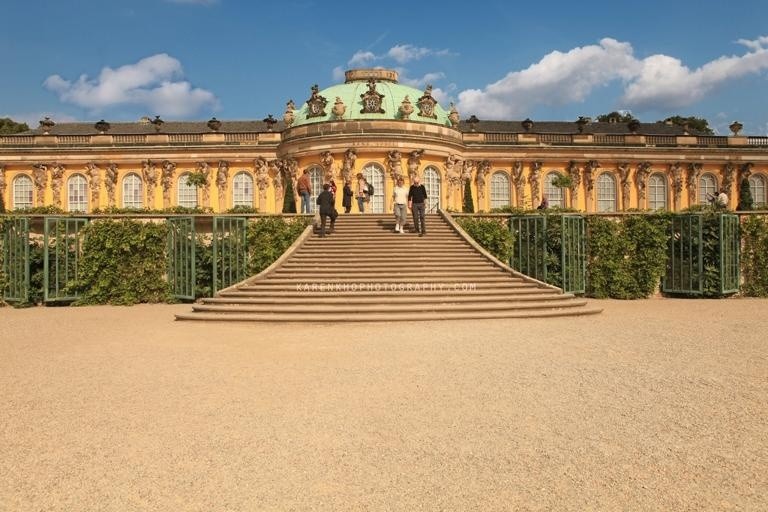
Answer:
[395,225,400,232]
[400,229,404,233]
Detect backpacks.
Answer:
[364,178,374,195]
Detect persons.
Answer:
[327,178,338,209]
[296,168,312,214]
[408,177,427,236]
[715,187,729,209]
[316,184,338,238]
[391,178,409,234]
[535,193,548,209]
[705,190,718,203]
[342,179,353,215]
[357,173,370,214]
